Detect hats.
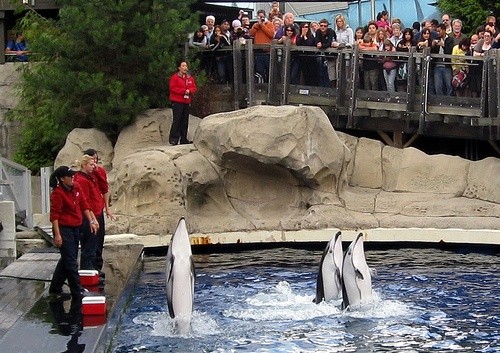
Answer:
[56,165,78,178]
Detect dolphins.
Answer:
[313,231,344,307]
[165,216,197,337]
[341,233,377,312]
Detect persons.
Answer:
[73,149,113,280]
[5,30,32,61]
[169,60,197,145]
[49,166,97,299]
[190,2,500,107]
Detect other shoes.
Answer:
[180,139,193,144]
[48,287,71,301]
[99,271,106,278]
[169,140,178,145]
[70,294,86,312]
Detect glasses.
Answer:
[442,19,448,22]
[320,24,327,27]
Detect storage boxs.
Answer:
[79,270,100,285]
[82,296,106,313]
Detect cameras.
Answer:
[260,16,265,21]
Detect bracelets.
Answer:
[89,221,94,224]
